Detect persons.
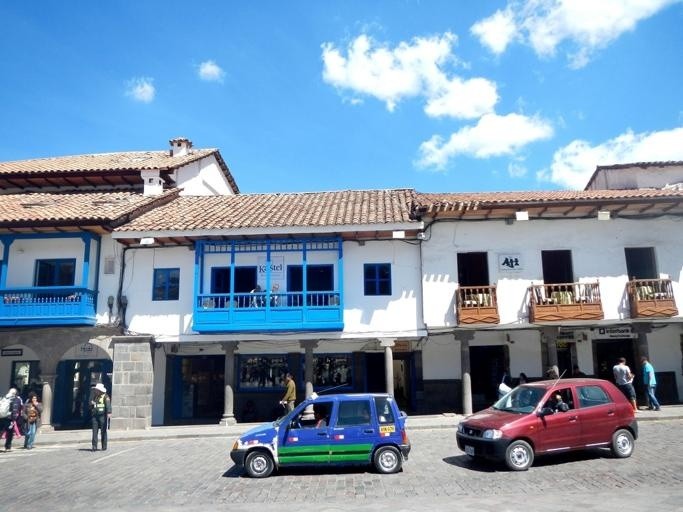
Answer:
[89,383,111,451]
[514,366,585,415]
[639,356,661,411]
[311,404,328,428]
[281,373,296,412]
[0,386,42,451]
[612,357,641,412]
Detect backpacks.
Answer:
[9,397,21,412]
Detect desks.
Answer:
[478,293,489,305]
[636,286,655,300]
[550,291,573,305]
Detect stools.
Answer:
[464,300,477,305]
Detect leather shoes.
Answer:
[645,407,654,410]
[653,406,661,411]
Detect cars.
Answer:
[231,381,410,479]
[455,371,638,472]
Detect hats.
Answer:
[92,384,107,393]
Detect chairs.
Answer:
[572,287,594,304]
[533,288,552,305]
[650,283,672,299]
